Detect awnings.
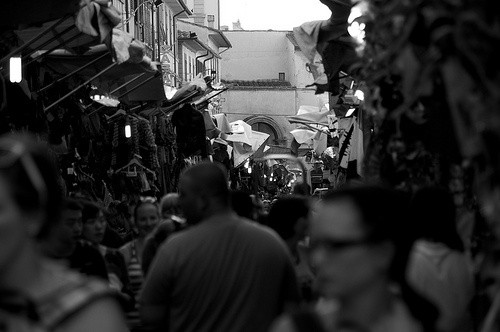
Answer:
[0,0,228,117]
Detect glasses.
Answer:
[309,234,371,253]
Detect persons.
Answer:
[0,130,500,332]
